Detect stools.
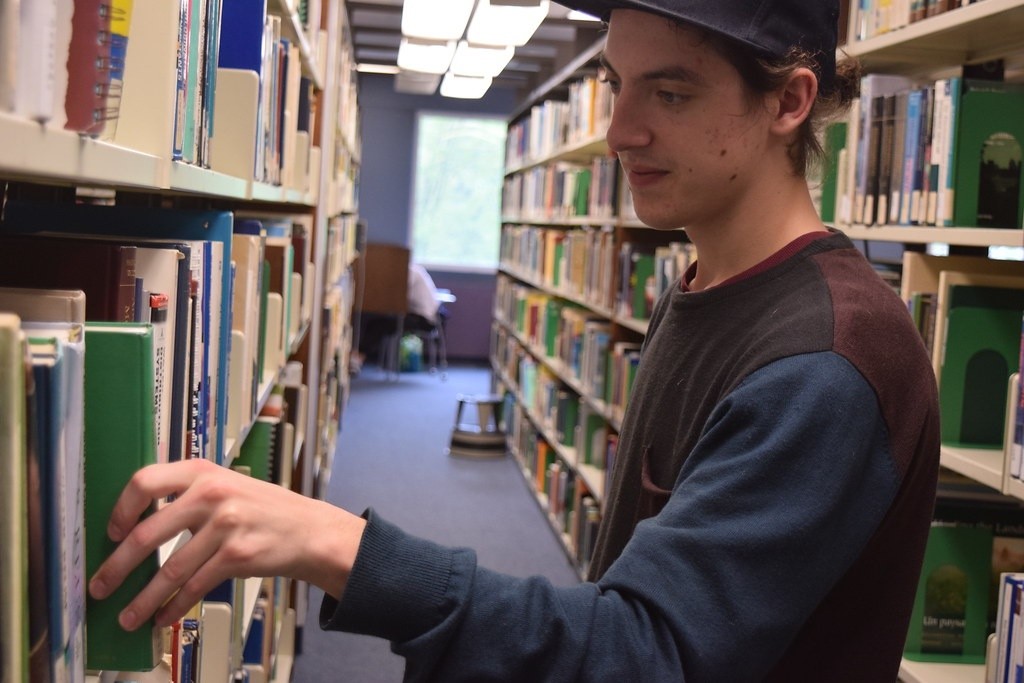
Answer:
[386,327,444,382]
[450,394,505,448]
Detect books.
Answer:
[487,0,1024,683]
[0,0,368,681]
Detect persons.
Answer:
[89,0,942,683]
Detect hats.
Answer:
[550,0,840,100]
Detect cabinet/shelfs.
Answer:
[489,0,1024,683]
[0,0,361,682]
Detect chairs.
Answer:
[355,243,412,382]
